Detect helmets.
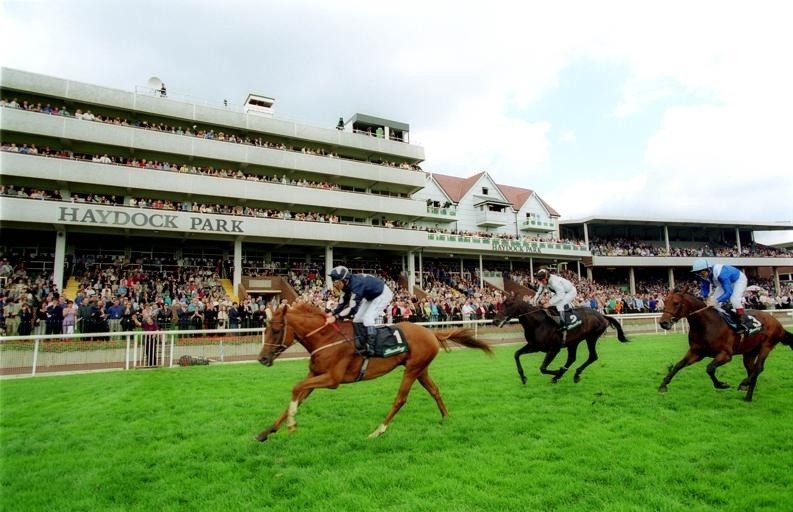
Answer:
[326,266,350,279]
[534,269,550,279]
[691,259,712,272]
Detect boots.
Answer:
[735,309,748,337]
[354,326,376,356]
[557,310,566,333]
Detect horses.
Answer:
[656,286,793,403]
[491,290,631,385]
[254,302,492,442]
